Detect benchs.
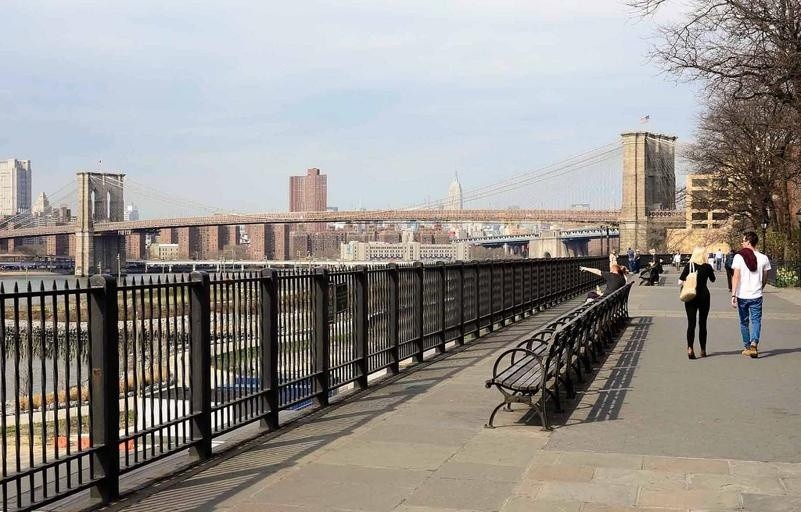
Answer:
[483,280,635,431]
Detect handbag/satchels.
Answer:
[679,262,698,302]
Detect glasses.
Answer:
[741,239,747,243]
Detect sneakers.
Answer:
[688,346,695,359]
[700,348,706,357]
[742,349,751,356]
[749,342,758,358]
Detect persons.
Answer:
[730,230,773,356]
[724,249,736,292]
[578,247,722,305]
[543,250,551,258]
[678,247,716,359]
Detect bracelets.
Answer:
[732,296,735,297]
